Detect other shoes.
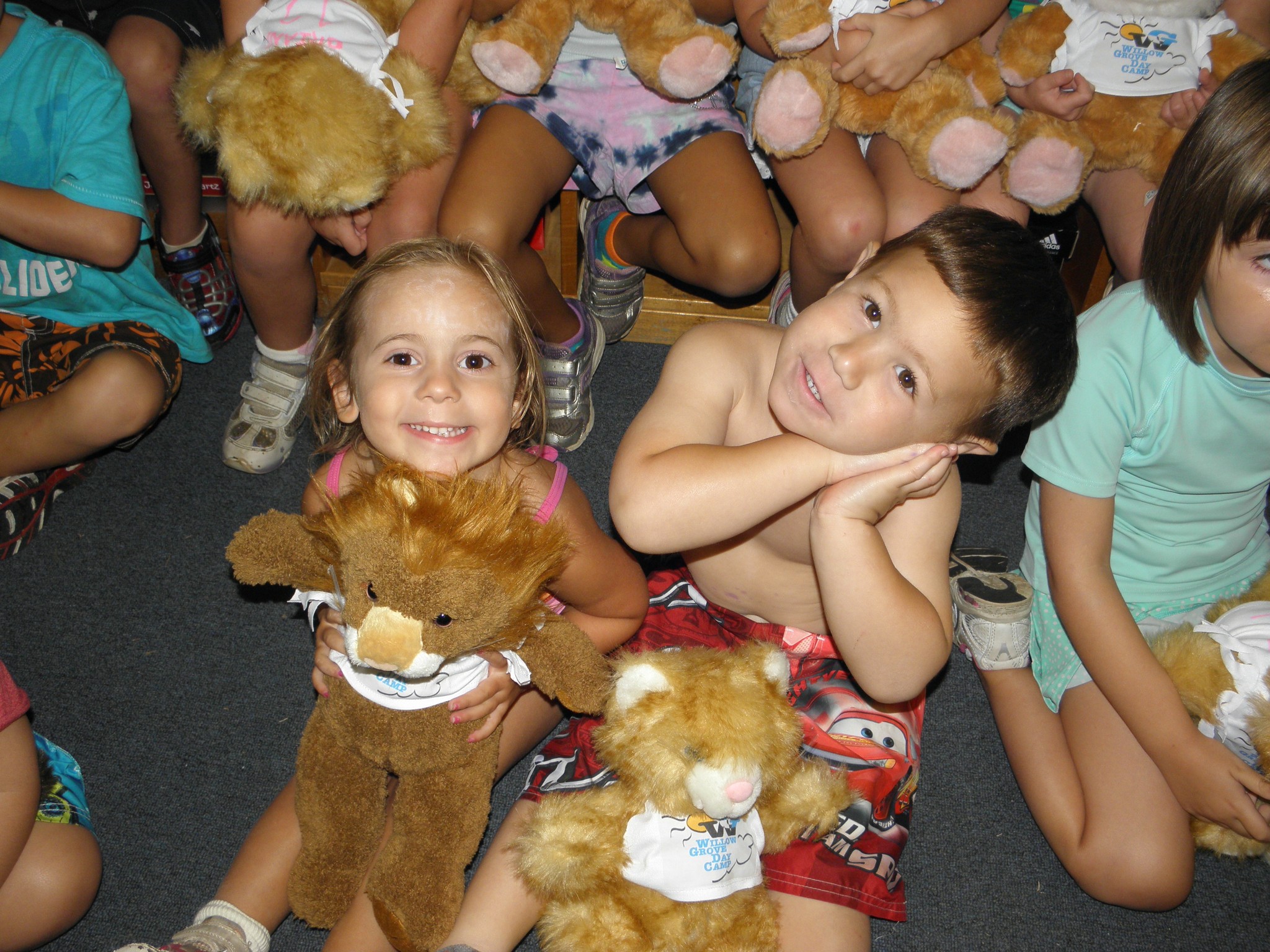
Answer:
[113,915,254,952]
[0,462,86,560]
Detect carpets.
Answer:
[2,311,1270,952]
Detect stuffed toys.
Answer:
[1151,571,1270,857]
[173,0,501,212]
[515,645,854,952]
[471,0,740,100]
[995,0,1270,214]
[752,0,1018,192]
[224,462,614,952]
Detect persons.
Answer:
[0,658,101,952]
[117,236,649,952]
[449,207,1079,952]
[0,0,1270,569]
[951,60,1270,908]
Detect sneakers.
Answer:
[770,271,795,329]
[534,296,606,454]
[943,543,1037,670]
[154,211,244,348]
[578,195,647,343]
[220,330,321,475]
